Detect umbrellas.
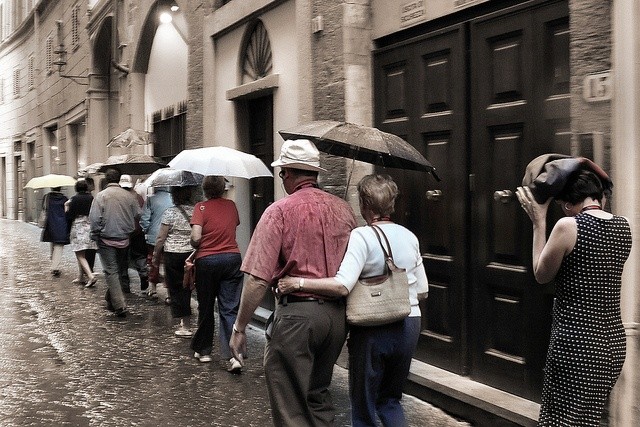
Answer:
[98,153,165,189]
[278,120,441,200]
[78,158,122,182]
[143,165,205,201]
[23,173,77,202]
[170,143,275,179]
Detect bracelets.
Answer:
[229,320,249,340]
[295,276,305,296]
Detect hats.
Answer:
[270,139,330,173]
[118,174,133,188]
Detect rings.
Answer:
[514,198,538,208]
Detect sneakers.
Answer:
[193,352,213,363]
[229,354,244,371]
[175,329,192,339]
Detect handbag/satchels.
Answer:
[38,194,48,228]
[182,250,196,291]
[345,223,411,325]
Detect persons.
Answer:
[64,176,97,288]
[152,185,202,338]
[140,174,176,306]
[276,176,431,235]
[516,167,633,423]
[88,170,138,318]
[228,140,359,423]
[40,181,68,278]
[190,176,241,373]
[115,173,147,297]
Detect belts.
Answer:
[278,294,319,302]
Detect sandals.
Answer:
[164,297,171,305]
[147,292,159,300]
[85,276,99,287]
[72,277,83,283]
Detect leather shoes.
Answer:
[115,307,129,316]
